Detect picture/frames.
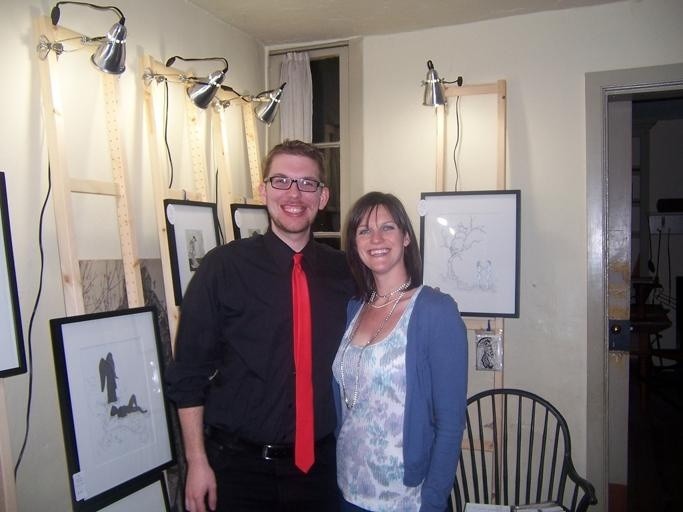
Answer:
[49,305,176,512]
[0,173,27,379]
[434,78,509,192]
[419,189,519,319]
[163,198,220,306]
[230,203,271,241]
[457,323,504,452]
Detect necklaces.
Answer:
[339,276,411,410]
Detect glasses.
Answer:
[264,175,325,192]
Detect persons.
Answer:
[166,139,361,511]
[327,189,468,512]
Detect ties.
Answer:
[291,253,316,475]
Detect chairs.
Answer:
[440,389,598,511]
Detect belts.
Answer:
[205,424,292,461]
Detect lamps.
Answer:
[50,2,127,77]
[253,82,285,122]
[166,54,228,108]
[420,59,463,105]
